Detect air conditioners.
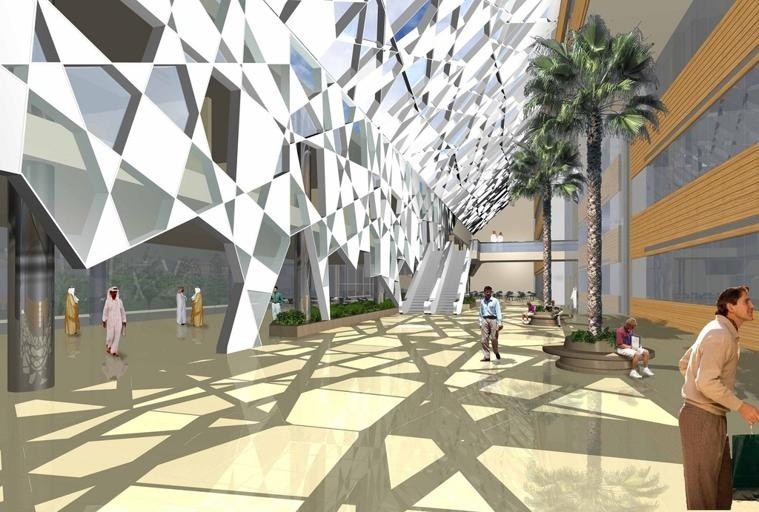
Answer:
[506,16,670,375]
